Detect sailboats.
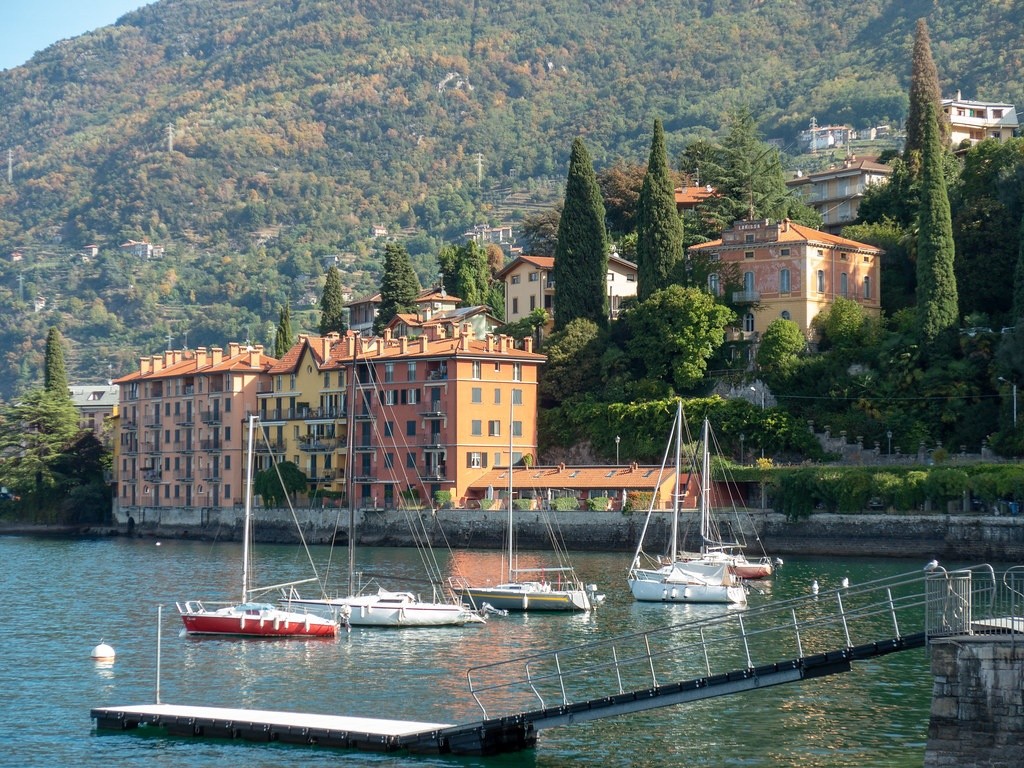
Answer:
[656,415,780,579]
[444,391,607,612]
[275,330,511,627]
[624,399,752,606]
[173,413,350,638]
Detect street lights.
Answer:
[740,433,745,463]
[614,435,620,465]
[886,430,893,464]
[750,386,765,458]
[998,376,1018,428]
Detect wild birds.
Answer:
[924,559,939,573]
[842,577,849,590]
[813,580,819,597]
